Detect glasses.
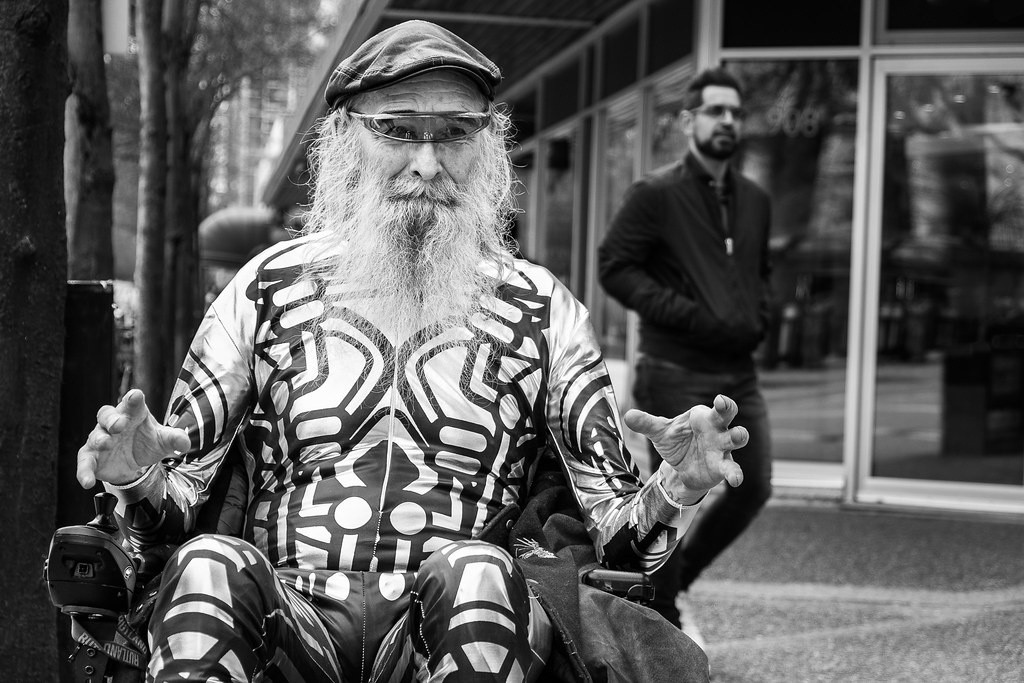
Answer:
[688,104,746,121]
[345,98,491,143]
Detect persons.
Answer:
[77,19,750,683]
[597,67,774,636]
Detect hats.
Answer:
[324,19,503,111]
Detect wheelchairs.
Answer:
[42,492,657,683]
